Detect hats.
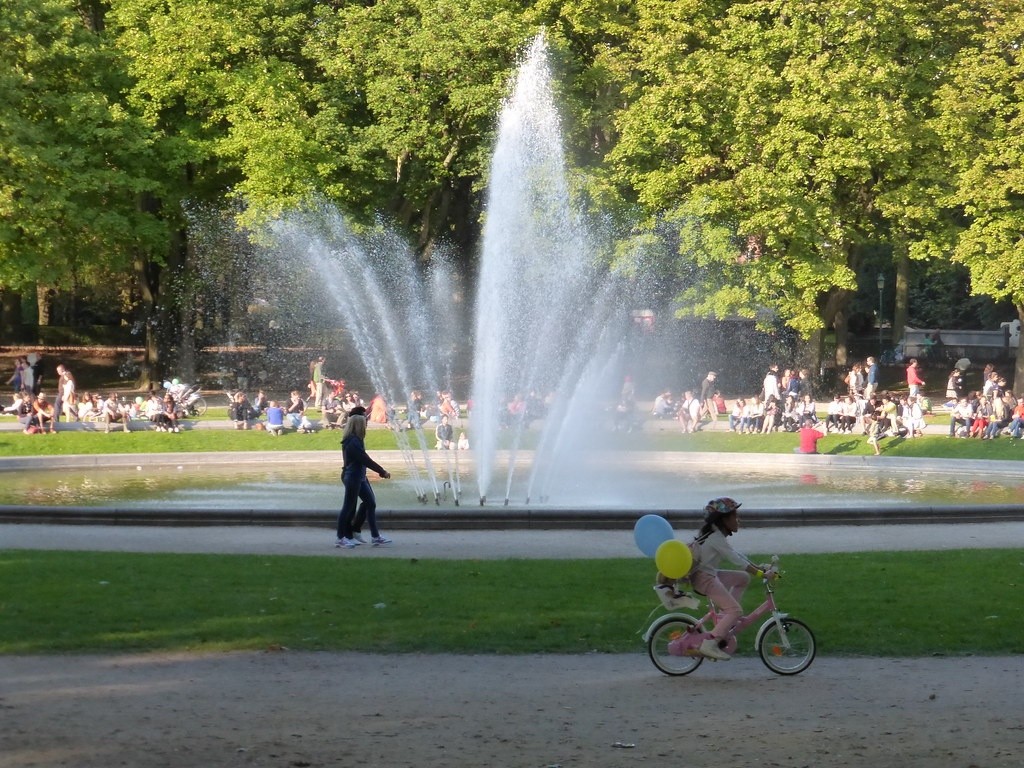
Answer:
[703,497,743,519]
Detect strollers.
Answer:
[168,383,202,417]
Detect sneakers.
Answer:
[336,537,355,548]
[355,534,368,543]
[372,537,392,545]
[345,538,361,544]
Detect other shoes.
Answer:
[875,444,881,455]
[700,639,732,661]
[613,425,1023,441]
[24,423,459,437]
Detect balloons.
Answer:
[635,514,676,561]
[656,539,694,578]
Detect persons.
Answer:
[336,415,390,546]
[689,496,776,662]
[3,350,1024,458]
[349,407,393,544]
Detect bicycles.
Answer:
[188,397,208,417]
[641,556,816,676]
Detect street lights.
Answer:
[877,273,885,344]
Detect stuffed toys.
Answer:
[656,571,685,599]
